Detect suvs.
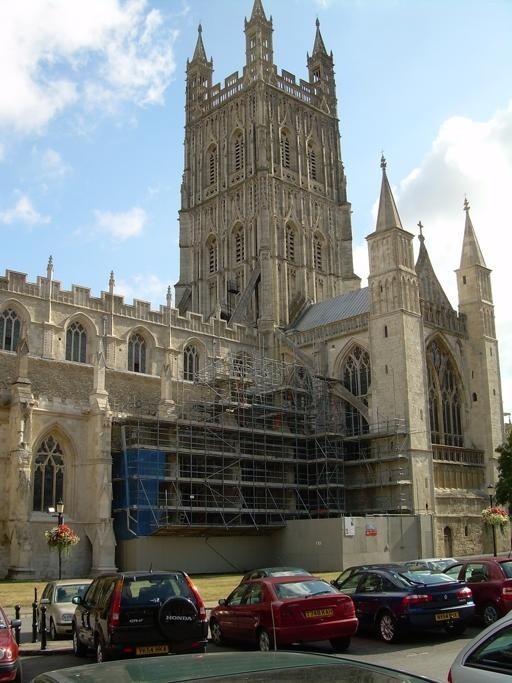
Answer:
[72,569,208,663]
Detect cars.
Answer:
[331,557,512,644]
[0,605,22,682]
[30,649,441,683]
[448,610,512,683]
[211,567,359,652]
[35,579,95,641]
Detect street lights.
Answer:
[487,483,497,556]
[56,497,65,582]
